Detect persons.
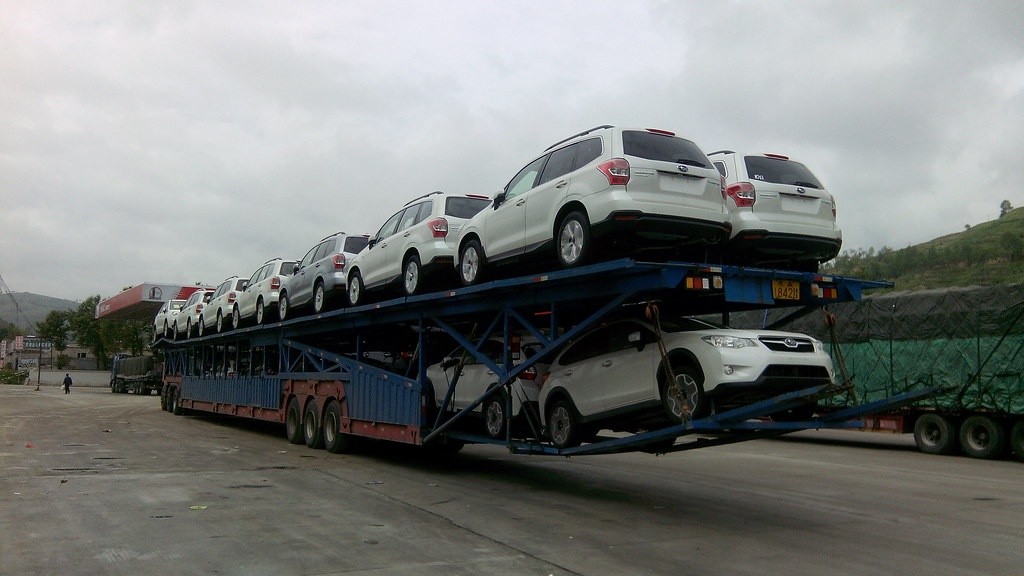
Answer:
[63,373,72,394]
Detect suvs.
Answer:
[538,312,839,451]
[415,337,555,441]
[278,231,371,321]
[172,290,215,341]
[152,299,186,343]
[706,148,843,273]
[232,258,302,328]
[345,191,493,308]
[197,275,250,337]
[208,331,418,376]
[453,123,733,286]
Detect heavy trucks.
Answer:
[687,281,1024,463]
[109,353,165,396]
[149,255,961,458]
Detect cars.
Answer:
[18,358,37,367]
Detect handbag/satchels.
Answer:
[61,384,66,390]
